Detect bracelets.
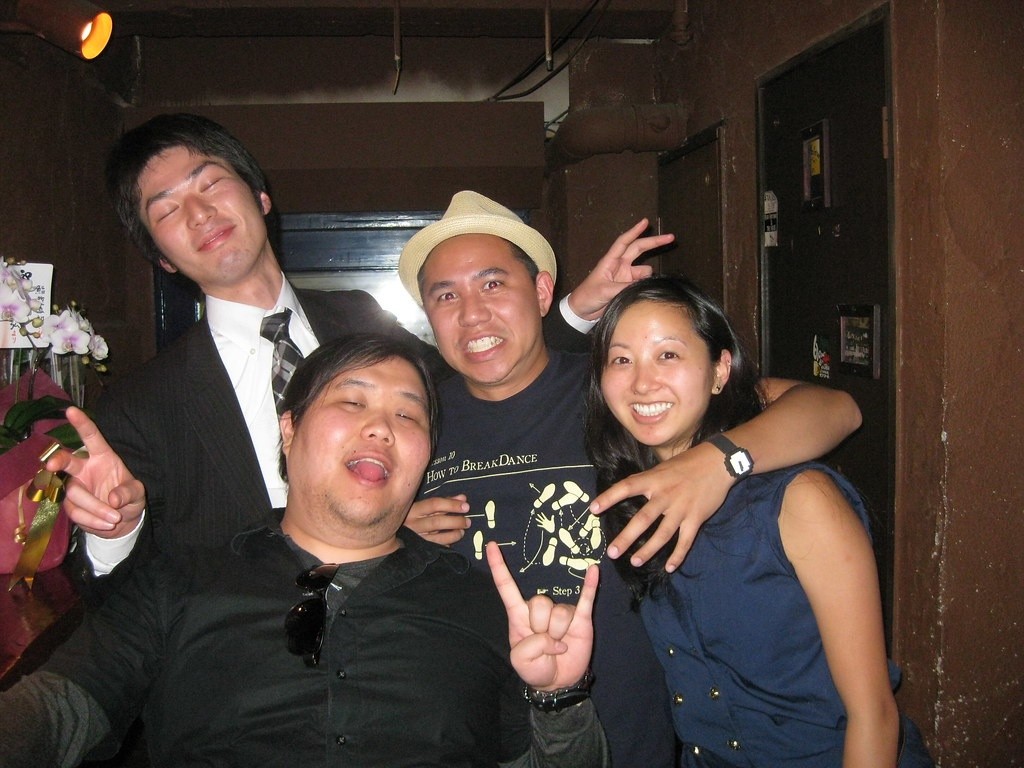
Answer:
[522,669,595,712]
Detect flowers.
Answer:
[1,257,112,458]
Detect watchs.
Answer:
[704,431,754,480]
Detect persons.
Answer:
[581,271,946,767]
[404,185,868,766]
[42,103,675,614]
[1,324,616,766]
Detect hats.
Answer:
[398,190,556,306]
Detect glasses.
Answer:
[283,563,340,669]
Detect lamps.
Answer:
[76,10,113,60]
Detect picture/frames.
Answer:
[833,303,880,378]
[800,119,831,211]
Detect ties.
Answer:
[258,309,305,419]
